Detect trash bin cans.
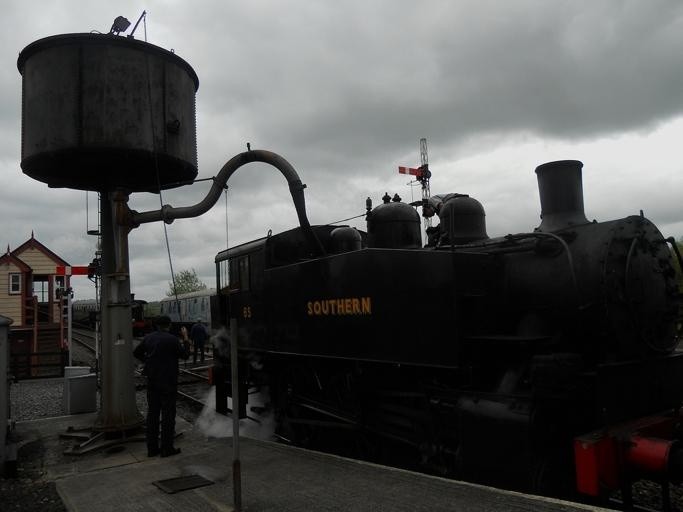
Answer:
[64,366,91,415]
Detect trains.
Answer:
[213,159,683,512]
[71,289,217,346]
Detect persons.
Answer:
[191,318,209,364]
[133,314,191,459]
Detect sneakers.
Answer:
[147,447,160,457]
[160,446,182,458]
